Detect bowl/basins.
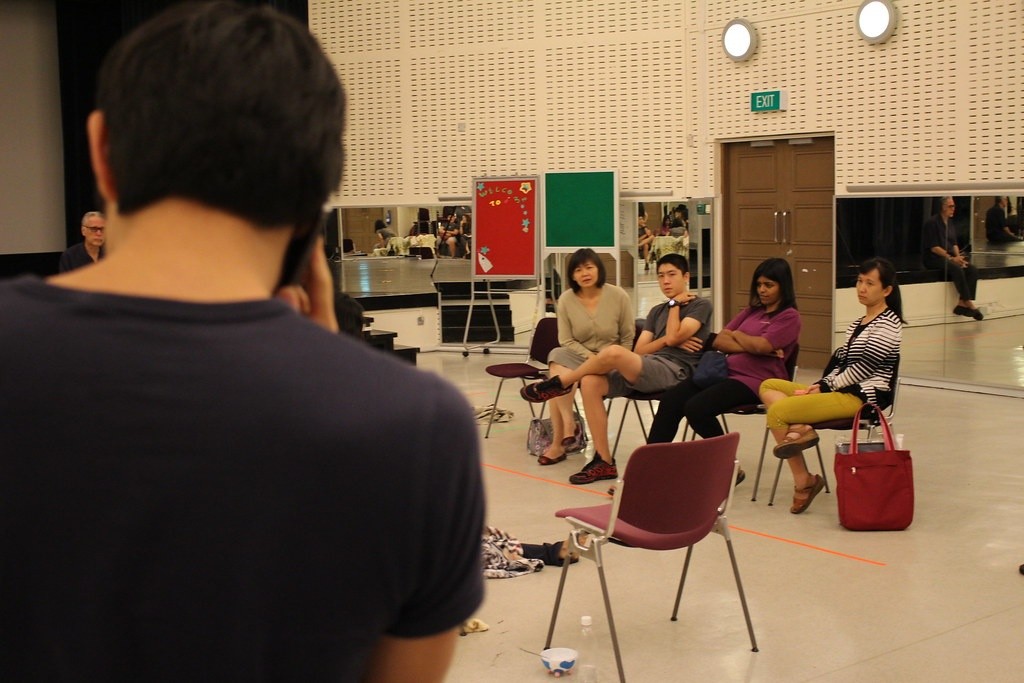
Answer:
[540,647,578,677]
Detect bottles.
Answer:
[577,616,599,683]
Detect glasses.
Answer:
[83,225,104,233]
[949,205,955,208]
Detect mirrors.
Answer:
[972,196,1024,254]
[638,199,714,301]
[829,195,1024,396]
[339,203,477,294]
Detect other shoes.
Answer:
[971,309,983,320]
[608,486,617,495]
[561,425,580,446]
[953,305,973,317]
[736,470,745,486]
[538,453,566,465]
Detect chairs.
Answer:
[545,433,760,683]
[485,319,900,506]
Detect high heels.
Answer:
[772,424,819,459]
[791,474,825,514]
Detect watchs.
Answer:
[667,300,680,309]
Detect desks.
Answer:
[649,236,688,261]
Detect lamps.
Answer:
[722,20,757,60]
[856,0,896,41]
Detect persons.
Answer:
[58,211,106,273]
[922,196,984,321]
[638,202,690,270]
[520,253,713,485]
[0,0,487,683]
[605,258,802,496]
[985,196,1024,242]
[374,208,471,259]
[759,258,908,515]
[537,248,635,465]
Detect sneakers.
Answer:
[520,375,575,403]
[569,451,618,484]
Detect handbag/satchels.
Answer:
[526,411,588,455]
[834,402,914,531]
[694,350,728,384]
